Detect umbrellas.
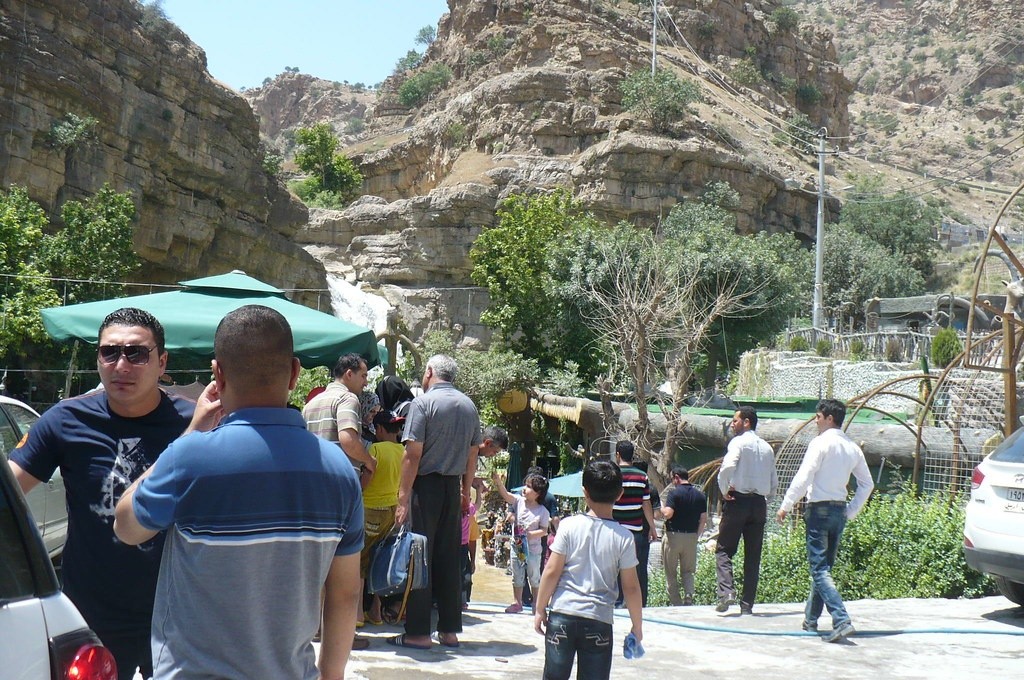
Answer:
[38,270,389,378]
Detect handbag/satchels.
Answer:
[368,521,428,602]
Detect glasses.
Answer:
[98,343,158,366]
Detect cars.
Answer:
[0,392,118,680]
[961,413,1024,606]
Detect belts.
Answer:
[666,529,697,533]
[809,501,846,507]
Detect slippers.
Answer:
[432,631,459,647]
[387,634,431,649]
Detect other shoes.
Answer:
[821,623,854,642]
[715,593,735,612]
[505,603,523,613]
[740,608,752,615]
[802,621,818,631]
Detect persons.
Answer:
[613,441,707,607]
[777,398,875,643]
[6,308,224,680]
[534,459,643,680]
[113,304,365,680]
[302,352,509,650]
[490,463,560,613]
[715,406,778,615]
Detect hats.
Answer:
[373,410,406,424]
